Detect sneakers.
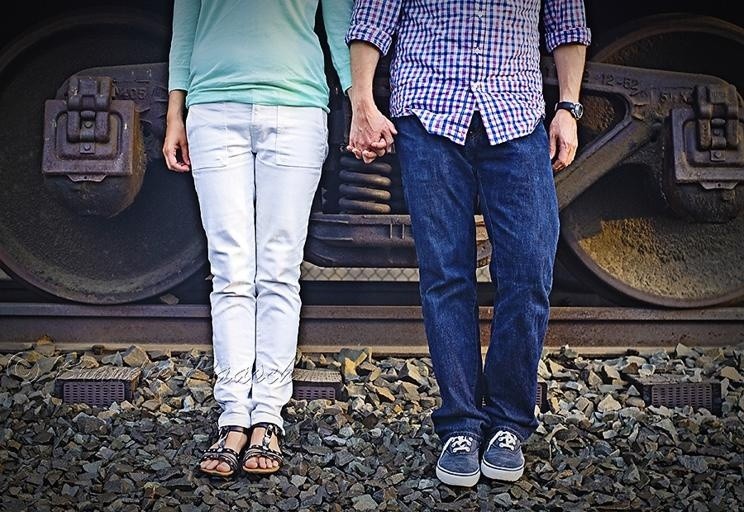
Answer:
[480,427,524,482]
[435,435,481,487]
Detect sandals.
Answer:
[198,424,249,476]
[241,421,282,474]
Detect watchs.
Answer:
[552,100,584,122]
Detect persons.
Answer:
[344,1,594,489]
[159,0,397,476]
